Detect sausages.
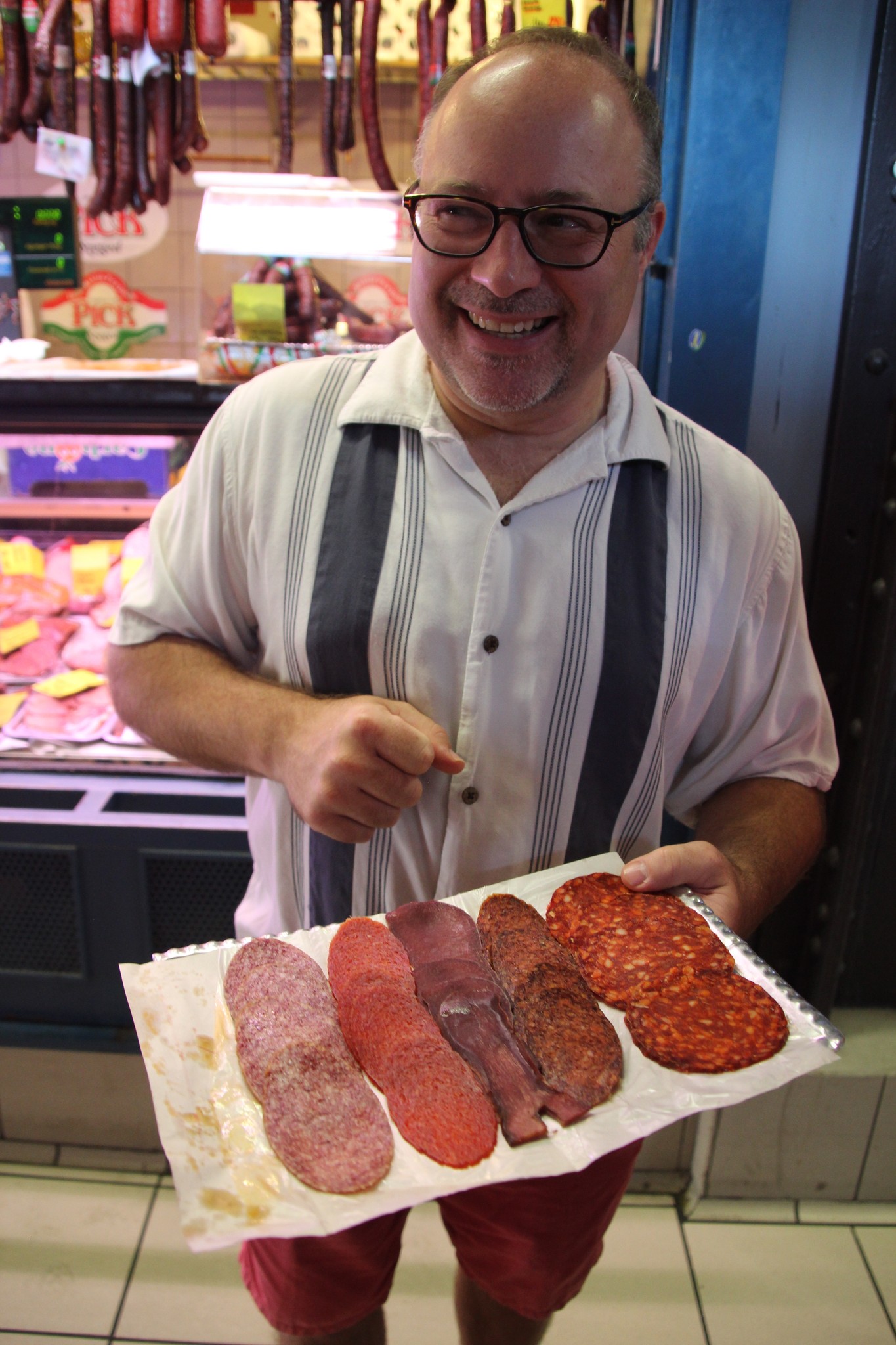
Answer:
[0,0,518,246]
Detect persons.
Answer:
[104,22,834,1344]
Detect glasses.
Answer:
[402,179,653,270]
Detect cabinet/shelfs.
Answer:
[0,355,275,1055]
